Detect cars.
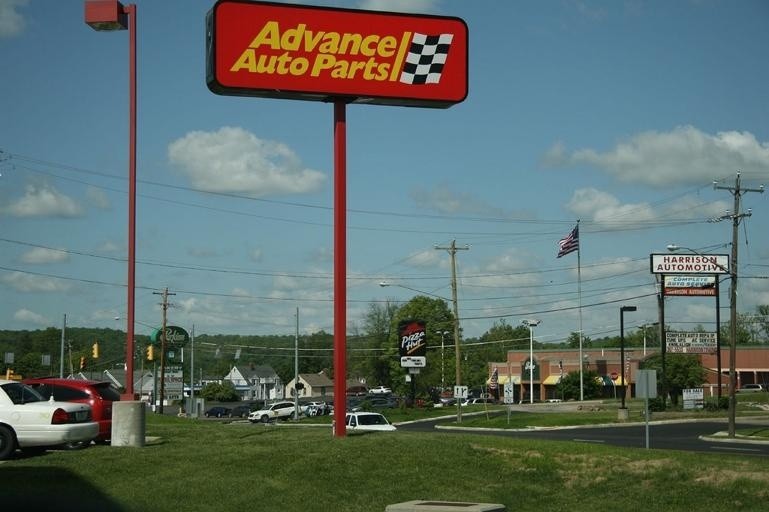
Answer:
[735,382,765,398]
[202,396,398,425]
[443,397,562,407]
[345,409,400,435]
[1,378,122,462]
[369,385,393,396]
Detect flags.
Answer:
[554,225,578,258]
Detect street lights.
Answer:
[666,242,738,278]
[378,280,456,303]
[113,313,166,338]
